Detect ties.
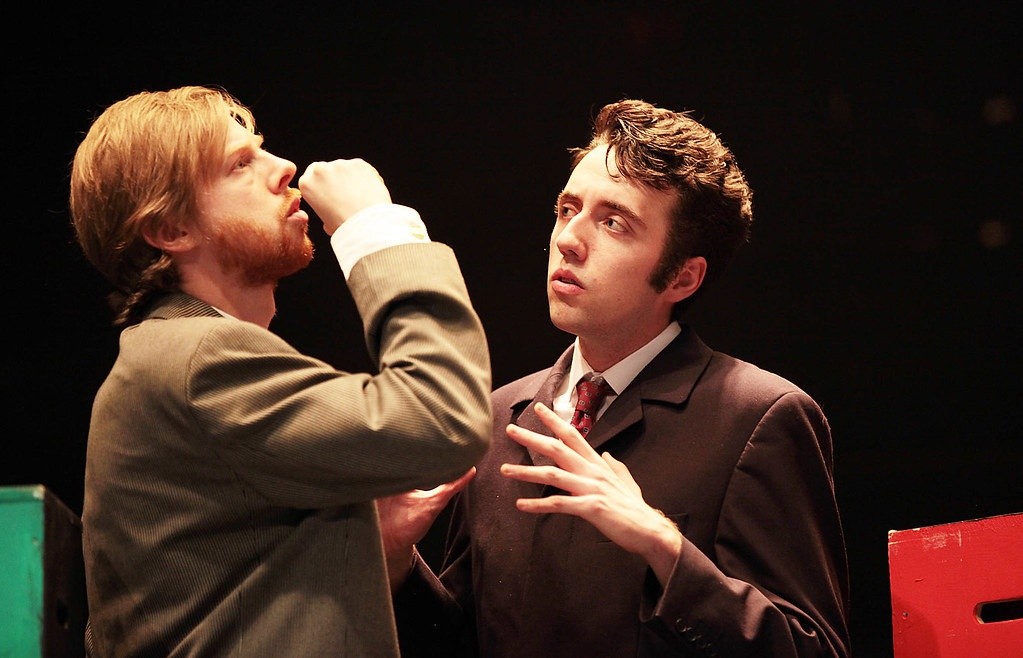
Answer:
[568,378,617,440]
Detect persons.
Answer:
[373,97,855,658]
[67,86,495,658]
